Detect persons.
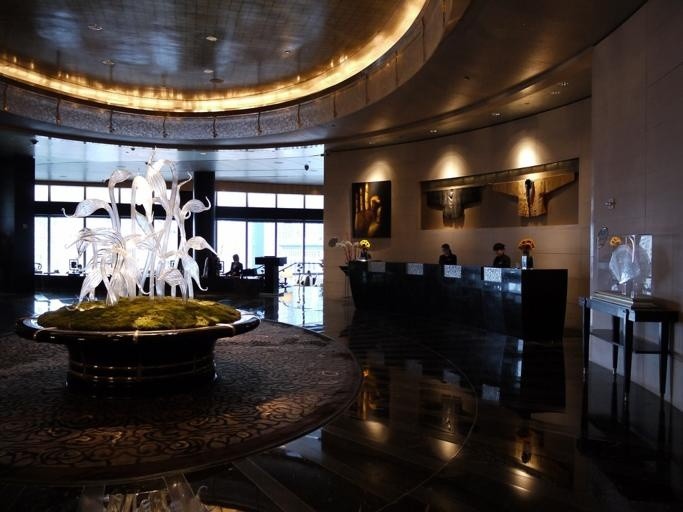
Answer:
[438,243,457,265]
[492,243,511,267]
[354,182,382,238]
[224,254,242,275]
[304,270,317,286]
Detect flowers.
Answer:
[517,239,535,256]
[359,239,370,249]
[609,236,620,247]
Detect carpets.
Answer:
[0,315,364,486]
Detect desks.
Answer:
[578,297,679,405]
